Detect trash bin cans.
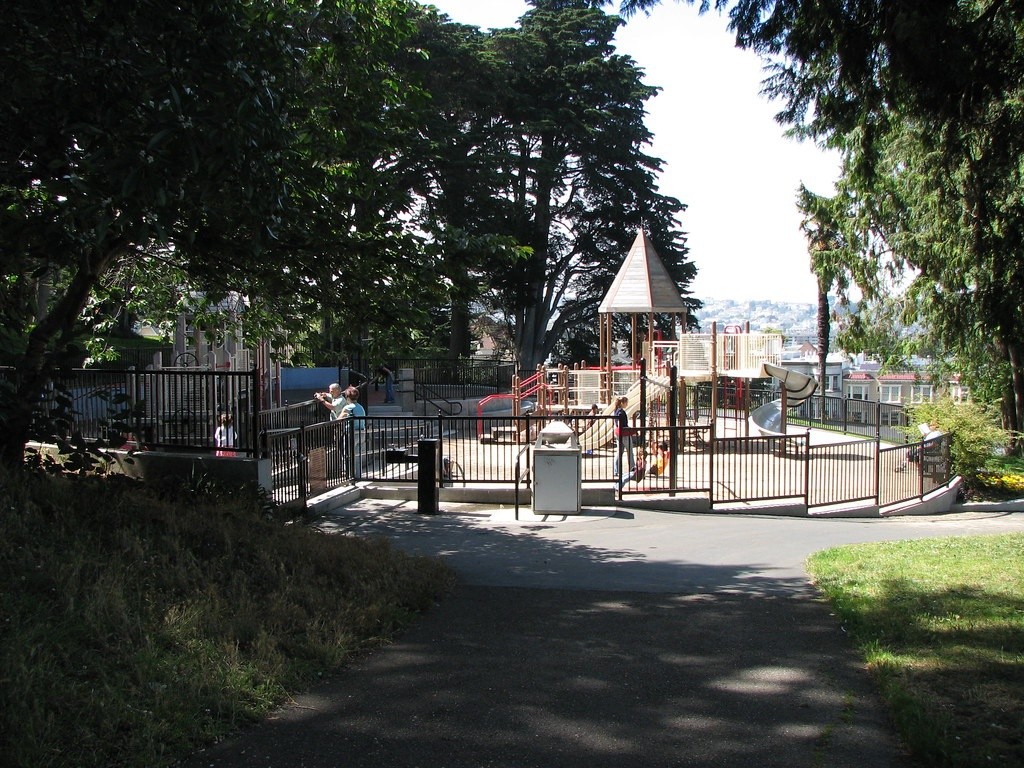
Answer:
[417,438,441,514]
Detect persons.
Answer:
[314,383,366,481]
[613,450,647,492]
[582,404,598,433]
[614,397,637,476]
[378,366,395,404]
[649,439,669,475]
[215,413,237,457]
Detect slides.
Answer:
[749,361,819,446]
[567,375,670,449]
